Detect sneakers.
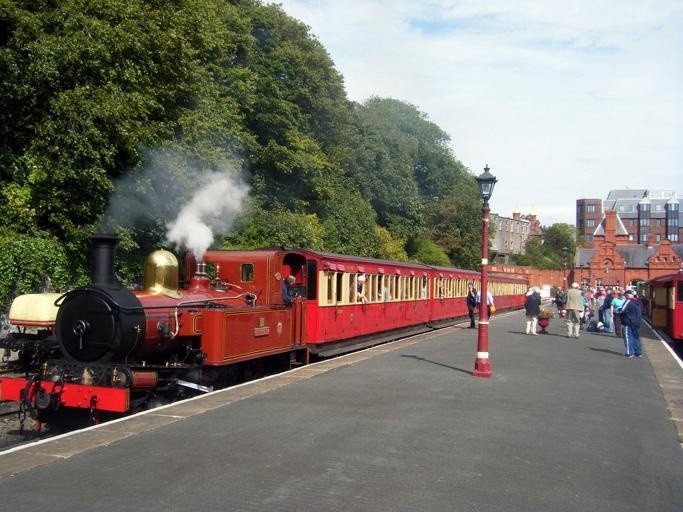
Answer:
[527,333,538,336]
[625,353,642,358]
[568,335,579,339]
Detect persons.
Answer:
[465,282,477,328]
[282,275,297,303]
[349,275,368,303]
[439,281,444,288]
[524,286,541,335]
[557,281,644,357]
[475,286,496,320]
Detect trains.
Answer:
[640,269,683,345]
[0,226,533,433]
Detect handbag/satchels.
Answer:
[489,305,495,313]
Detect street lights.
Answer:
[560,244,570,291]
[469,161,501,379]
[579,263,585,290]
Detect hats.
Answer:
[623,291,634,295]
